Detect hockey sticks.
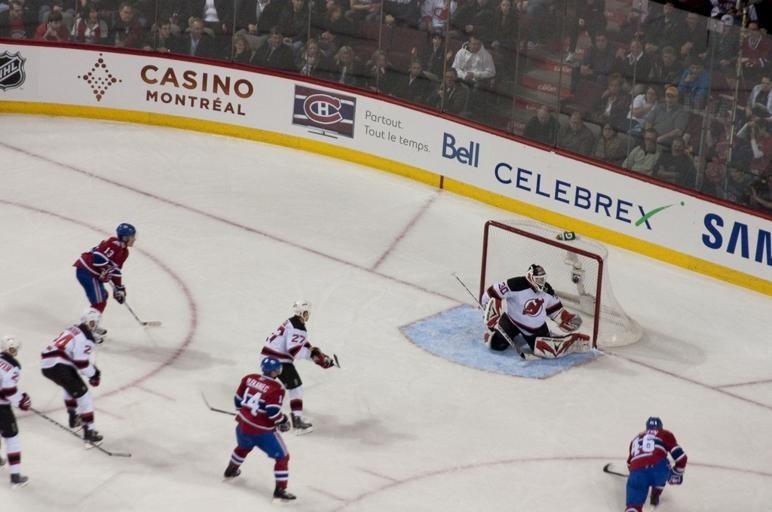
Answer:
[300,351,342,369]
[199,390,237,420]
[28,405,132,459]
[603,463,629,478]
[107,280,160,328]
[451,271,544,361]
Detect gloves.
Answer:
[279,414,290,431]
[88,366,101,387]
[19,393,30,410]
[485,298,503,330]
[552,308,582,331]
[667,468,683,485]
[309,348,334,369]
[113,285,126,304]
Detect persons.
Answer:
[709,13,741,80]
[512,0,546,50]
[182,15,219,58]
[570,29,614,98]
[388,58,436,104]
[70,3,109,45]
[142,16,186,55]
[361,48,397,96]
[452,35,497,89]
[677,12,708,60]
[259,302,335,430]
[591,72,633,130]
[221,33,253,65]
[736,73,772,134]
[294,38,331,80]
[722,163,754,208]
[72,222,137,344]
[481,263,591,359]
[310,2,353,57]
[556,110,595,156]
[621,127,661,177]
[748,118,772,176]
[623,415,688,511]
[39,306,103,443]
[647,45,683,100]
[186,0,232,36]
[0,336,32,484]
[642,2,683,49]
[223,355,297,501]
[709,0,758,28]
[589,121,627,166]
[736,21,772,81]
[34,10,70,42]
[490,0,519,84]
[731,107,763,166]
[683,153,716,199]
[269,0,308,49]
[625,85,660,135]
[38,0,65,24]
[355,0,401,60]
[565,0,607,63]
[344,0,369,25]
[0,0,34,40]
[522,104,560,145]
[449,0,495,41]
[644,86,690,144]
[104,2,144,49]
[417,0,458,33]
[423,33,454,80]
[652,135,694,187]
[614,39,651,84]
[327,46,363,87]
[237,0,281,37]
[748,167,772,213]
[677,56,709,109]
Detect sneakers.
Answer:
[70,420,82,427]
[0,458,5,467]
[84,430,102,441]
[96,327,107,335]
[272,488,294,498]
[93,335,103,343]
[11,474,28,482]
[225,469,240,477]
[292,415,312,430]
[649,496,659,507]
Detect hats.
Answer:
[666,86,681,99]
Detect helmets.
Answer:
[294,301,311,319]
[81,307,101,331]
[646,417,663,431]
[0,337,19,356]
[117,223,135,240]
[261,358,280,376]
[525,264,546,291]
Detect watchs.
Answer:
[167,49,170,55]
